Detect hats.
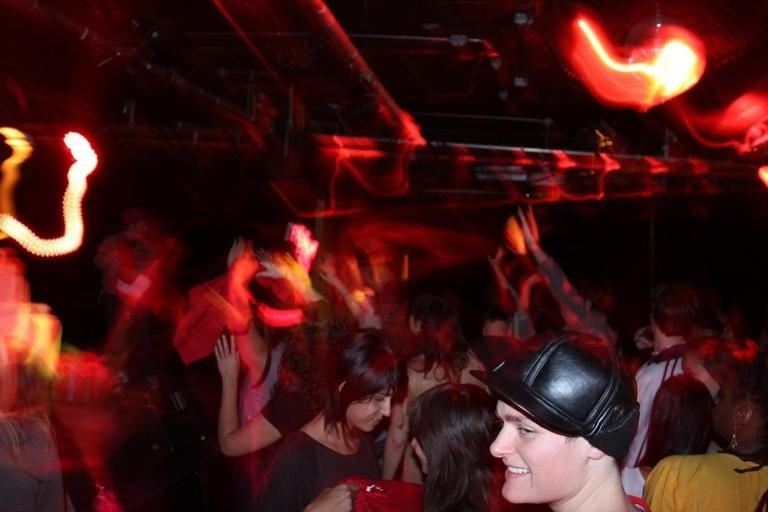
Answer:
[470,329,640,458]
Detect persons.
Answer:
[1,195,768,512]
